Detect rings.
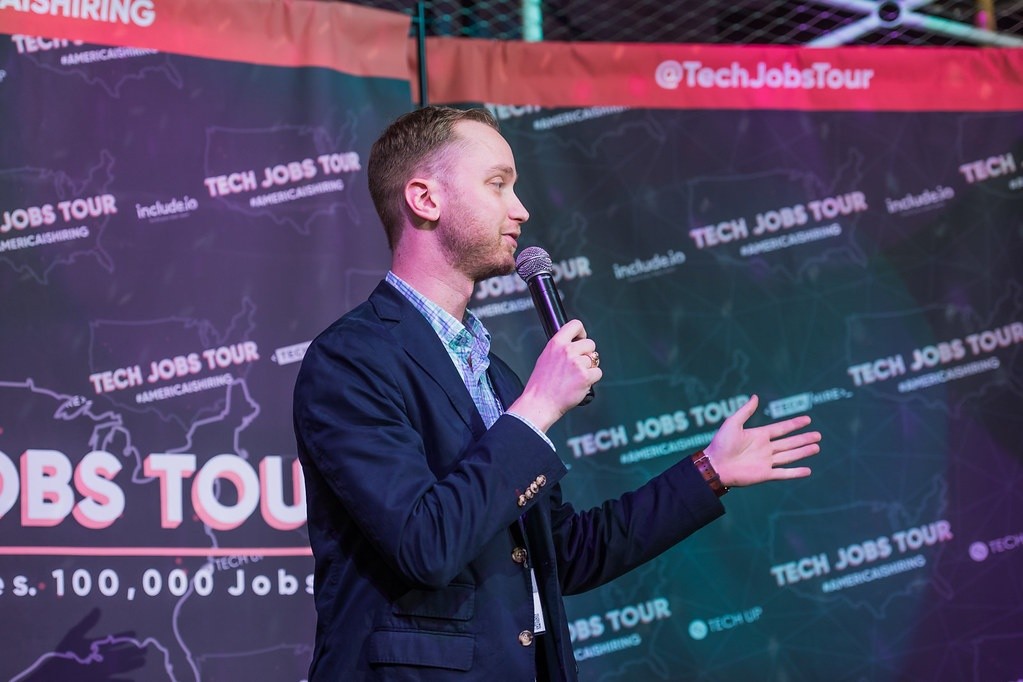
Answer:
[583,351,600,368]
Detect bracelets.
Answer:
[691,450,728,496]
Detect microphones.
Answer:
[515,246,596,407]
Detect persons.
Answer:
[292,105,822,682]
